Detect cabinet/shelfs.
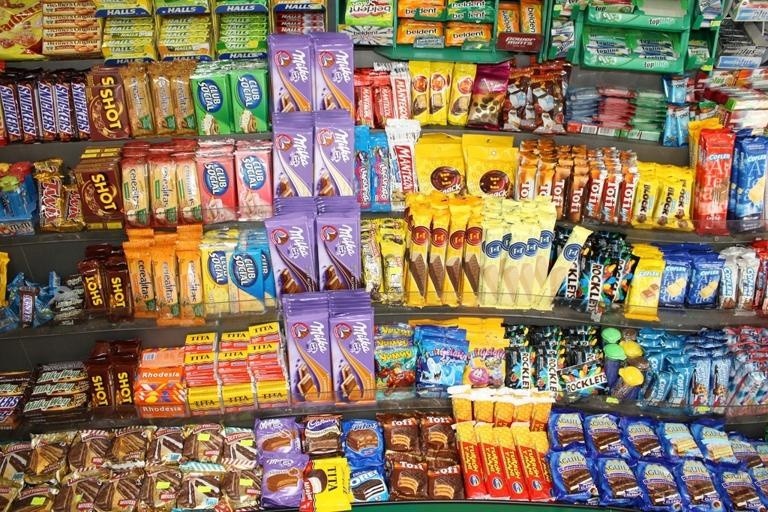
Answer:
[1,0,766,511]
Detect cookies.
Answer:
[408,253,567,302]
[262,429,461,499]
